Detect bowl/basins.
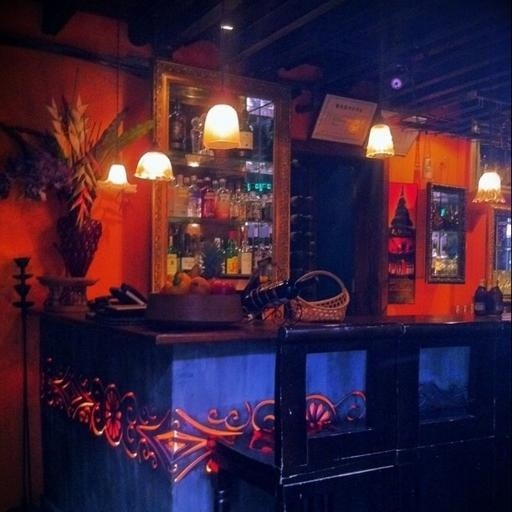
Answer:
[145,293,242,327]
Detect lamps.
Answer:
[203,0,240,148]
[96,1,137,194]
[365,56,396,160]
[135,1,175,183]
[472,105,506,204]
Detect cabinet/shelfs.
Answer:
[153,56,293,294]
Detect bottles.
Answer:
[167,95,272,275]
[242,276,321,310]
[473,279,504,316]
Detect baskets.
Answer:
[290,270,351,323]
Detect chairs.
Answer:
[212,321,405,512]
[402,323,511,512]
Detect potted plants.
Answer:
[0,74,160,276]
